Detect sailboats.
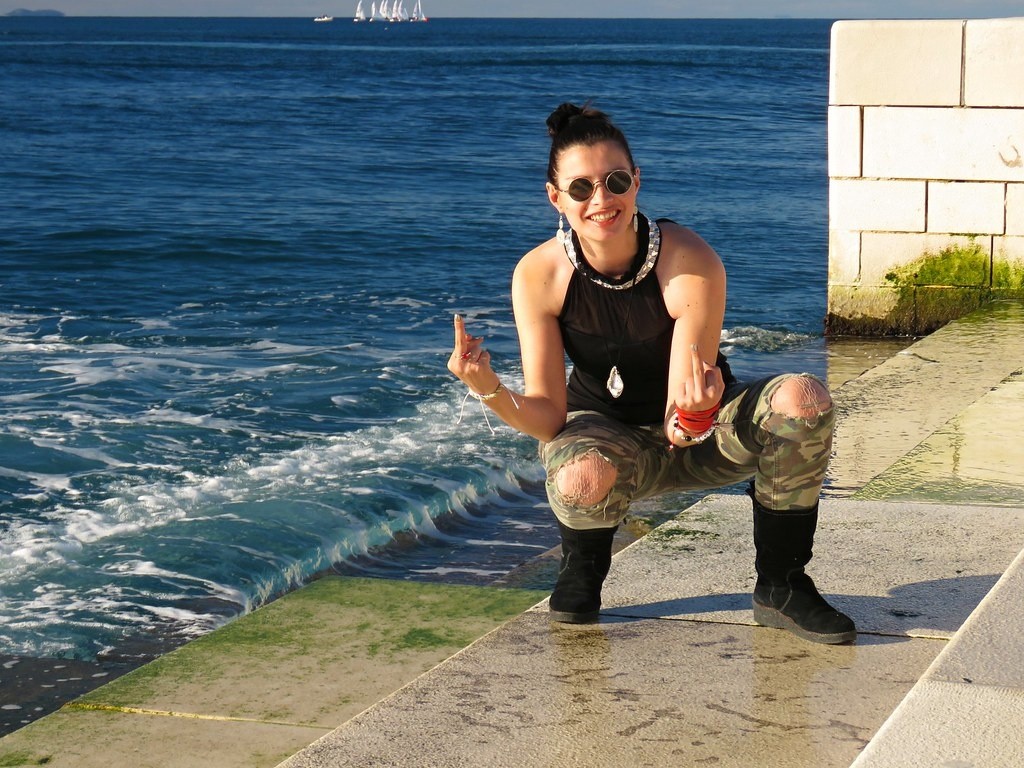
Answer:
[353,0,428,22]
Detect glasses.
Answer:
[555,169,637,201]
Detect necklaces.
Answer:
[593,258,637,398]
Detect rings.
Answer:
[460,353,467,359]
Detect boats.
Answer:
[314,15,333,21]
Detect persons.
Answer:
[445,100,856,644]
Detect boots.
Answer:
[745,480,857,644]
[549,513,627,622]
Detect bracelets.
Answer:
[668,401,720,452]
[456,384,519,435]
